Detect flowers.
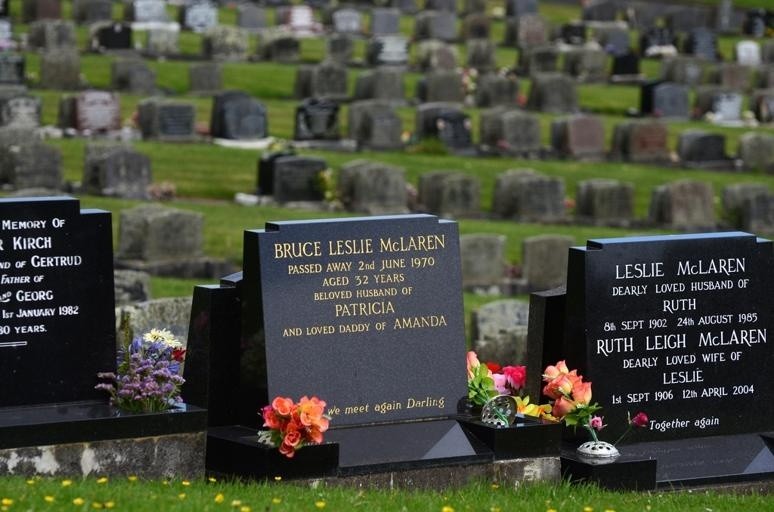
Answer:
[258,391,333,460]
[91,320,191,411]
[455,344,652,445]
